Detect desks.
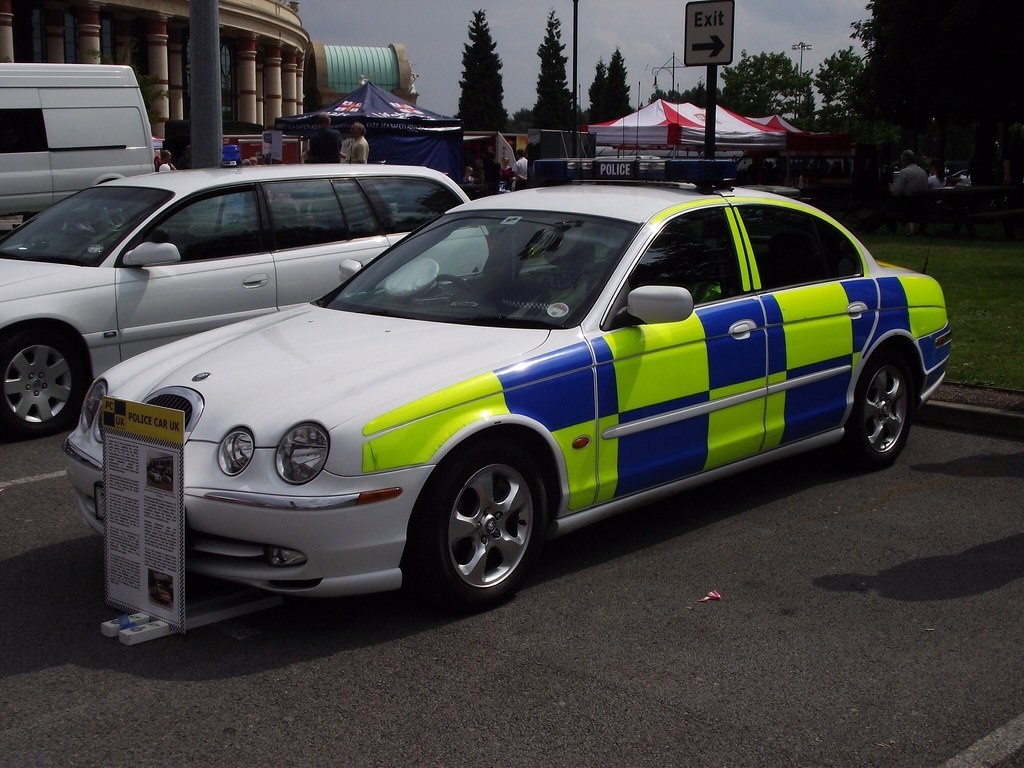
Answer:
[911,185,1024,240]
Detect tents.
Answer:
[274,80,465,183]
[579,99,851,187]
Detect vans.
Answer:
[0,62,157,217]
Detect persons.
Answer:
[155,144,192,171]
[408,59,419,93]
[884,129,1018,234]
[358,74,369,87]
[308,111,370,170]
[465,148,529,195]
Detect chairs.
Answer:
[542,239,596,318]
[144,199,193,256]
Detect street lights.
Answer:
[792,42,811,117]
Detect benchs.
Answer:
[951,208,1024,239]
[897,211,998,235]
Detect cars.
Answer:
[62,180,954,613]
[0,162,473,442]
[943,160,969,175]
[943,168,1000,187]
[877,164,901,182]
